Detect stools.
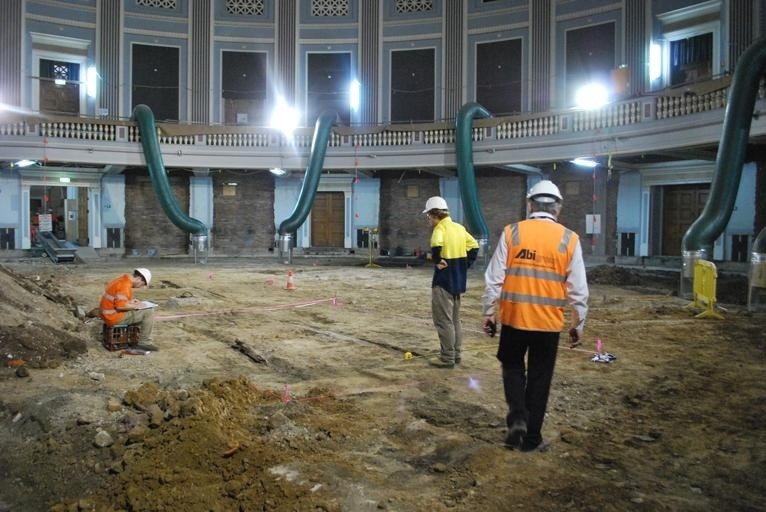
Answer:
[102,323,140,352]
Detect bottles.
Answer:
[286,271,294,289]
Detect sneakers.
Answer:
[136,343,160,352]
[455,357,461,363]
[426,356,455,369]
[520,433,544,453]
[503,420,527,448]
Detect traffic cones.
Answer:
[281,269,298,291]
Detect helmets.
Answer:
[421,194,449,215]
[134,267,152,286]
[526,179,564,204]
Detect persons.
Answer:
[97,267,160,353]
[482,178,591,453]
[420,195,482,370]
